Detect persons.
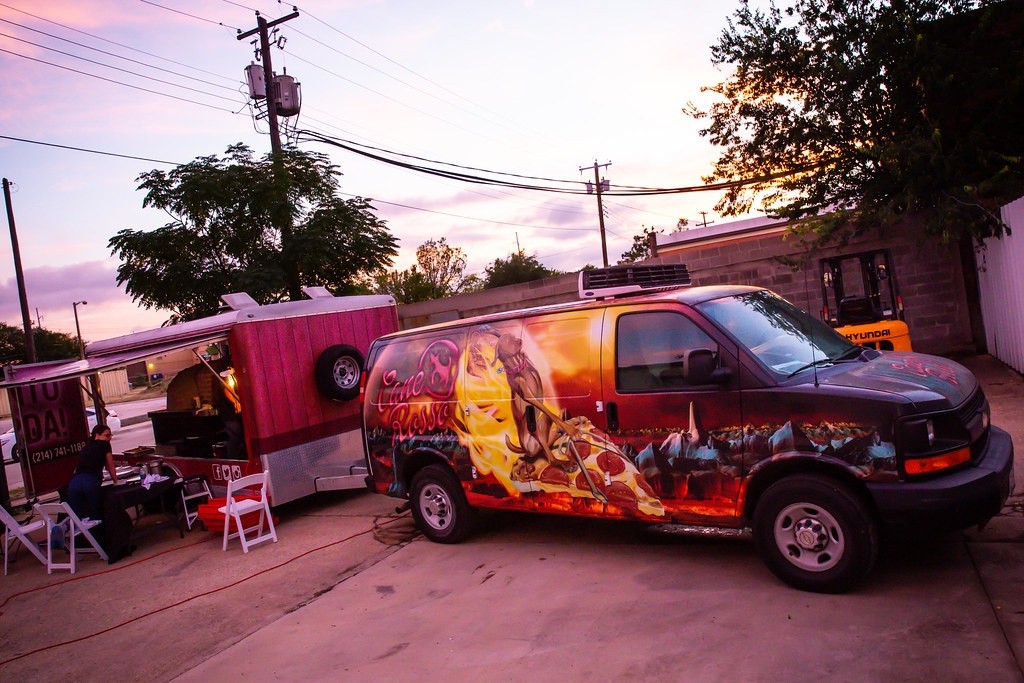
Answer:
[51,424,127,559]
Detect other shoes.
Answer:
[49,524,66,550]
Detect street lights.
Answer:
[73,300,89,360]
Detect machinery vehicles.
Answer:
[817,249,913,357]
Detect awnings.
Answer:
[0,330,240,403]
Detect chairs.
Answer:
[0,504,48,576]
[218,470,278,554]
[31,502,110,576]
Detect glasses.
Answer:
[104,434,113,438]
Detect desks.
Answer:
[100,474,181,554]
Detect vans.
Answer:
[360,262,1017,593]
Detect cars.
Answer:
[0,406,122,464]
